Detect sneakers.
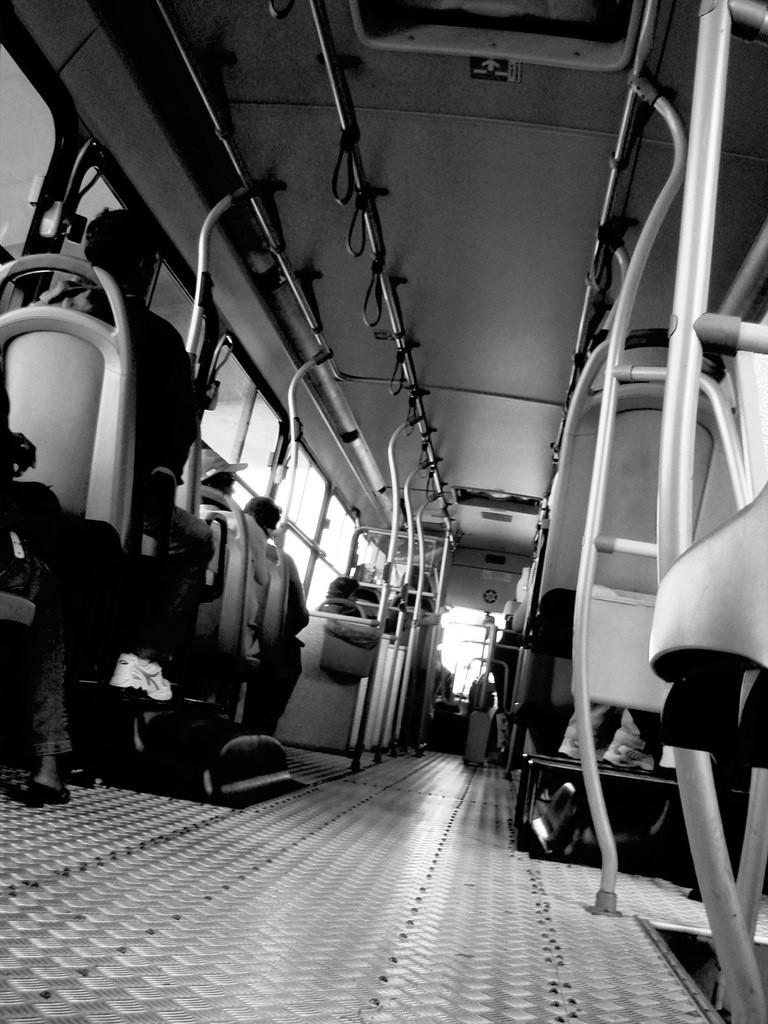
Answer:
[108,652,172,700]
[557,736,606,760]
[603,740,654,770]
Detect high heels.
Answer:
[25,777,71,807]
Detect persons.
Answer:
[198,447,309,737]
[28,209,217,703]
[0,360,73,806]
[316,565,436,743]
[492,599,768,903]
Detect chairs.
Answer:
[1,249,408,760]
[472,324,767,869]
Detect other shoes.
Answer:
[214,698,233,719]
[398,745,408,754]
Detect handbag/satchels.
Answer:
[320,618,381,677]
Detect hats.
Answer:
[326,577,358,598]
[242,497,281,529]
[182,449,248,483]
